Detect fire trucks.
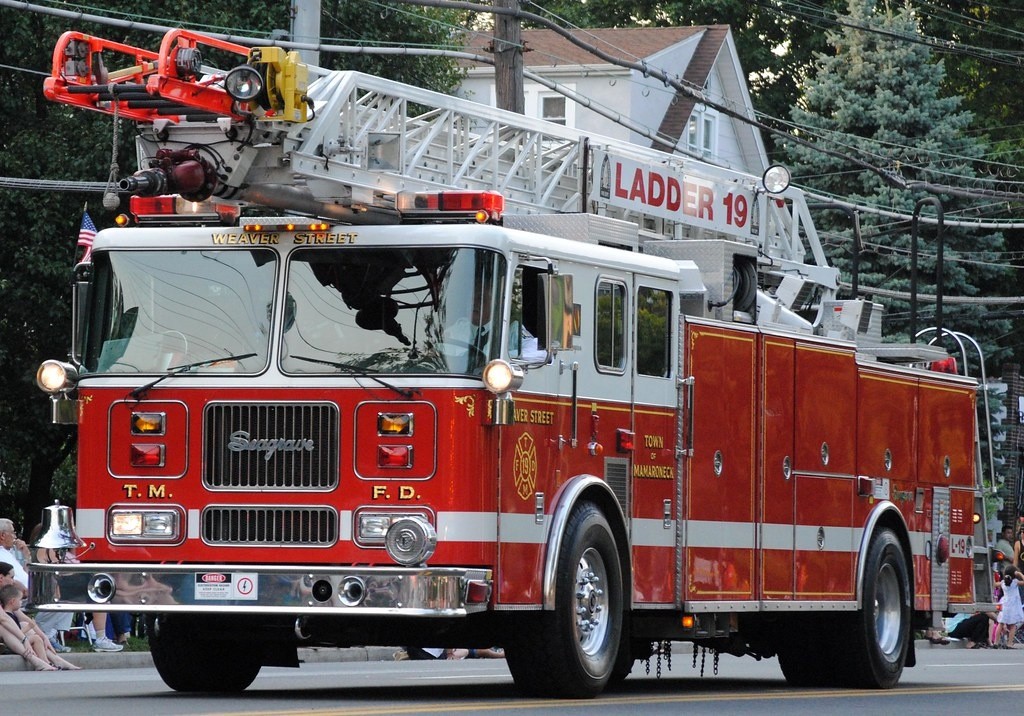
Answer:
[26,26,1005,701]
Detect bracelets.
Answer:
[21,636,27,644]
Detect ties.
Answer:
[467,327,489,371]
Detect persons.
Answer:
[428,268,547,374]
[27,522,145,652]
[0,561,83,671]
[993,565,1024,649]
[0,518,74,653]
[924,526,1024,649]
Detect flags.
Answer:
[72,212,99,271]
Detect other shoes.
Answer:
[117,631,131,643]
[966,642,1017,649]
[926,635,950,646]
[35,665,59,671]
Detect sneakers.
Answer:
[50,641,71,653]
[92,636,124,652]
